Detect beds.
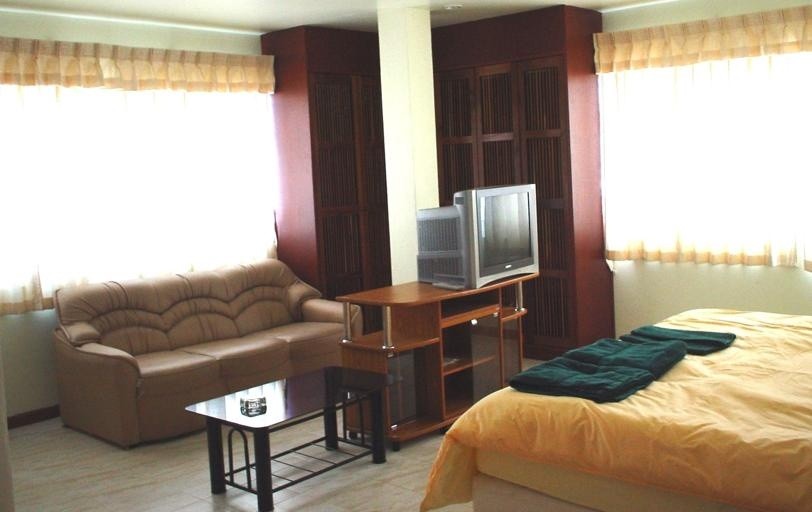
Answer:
[472,306,810,512]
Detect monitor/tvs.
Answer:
[415,181,539,291]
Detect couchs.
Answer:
[50,257,364,453]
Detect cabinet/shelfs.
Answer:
[335,272,540,454]
[259,23,393,336]
[431,4,615,362]
